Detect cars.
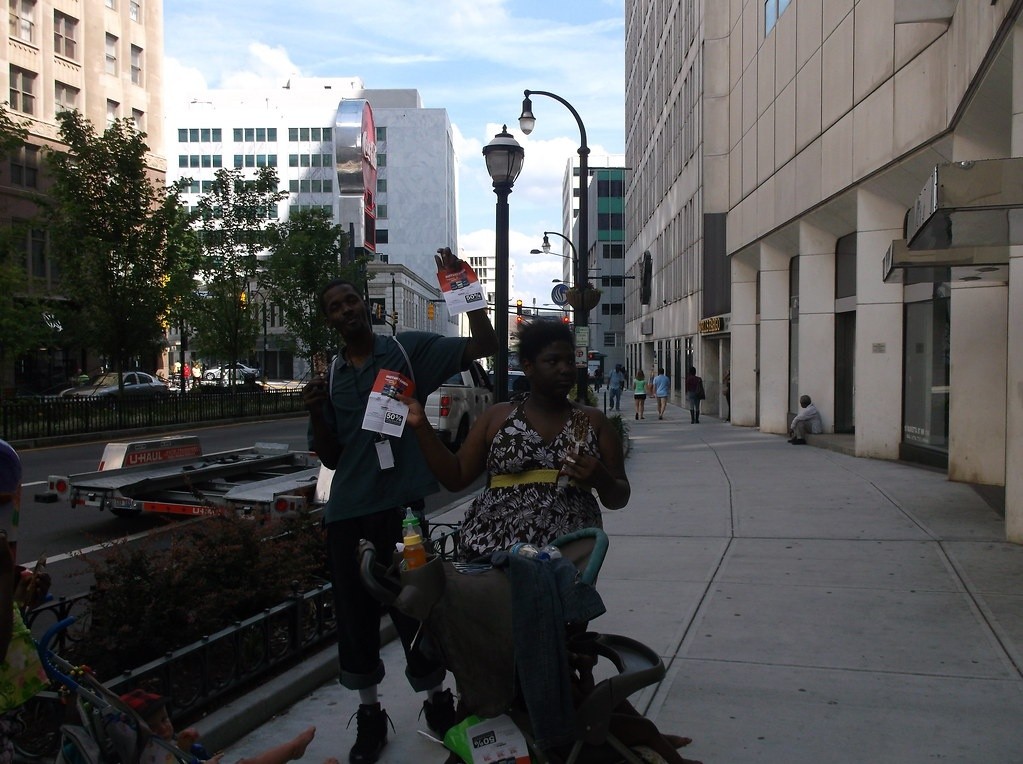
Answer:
[487,371,525,392]
[59,372,169,404]
[204,362,260,380]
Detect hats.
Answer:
[119,689,166,713]
[615,364,622,368]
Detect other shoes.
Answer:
[609,407,612,410]
[616,409,622,411]
[659,416,662,419]
[787,436,806,445]
[641,417,646,419]
[635,412,638,420]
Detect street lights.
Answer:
[481,127,523,402]
[390,270,396,336]
[518,90,589,405]
[531,232,578,328]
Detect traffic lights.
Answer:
[516,301,522,324]
[564,317,569,326]
[377,304,384,319]
[393,312,398,324]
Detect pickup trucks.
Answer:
[424,360,495,450]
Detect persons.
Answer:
[301,247,500,764]
[687,367,702,424]
[633,370,647,420]
[787,395,823,445]
[396,317,630,670]
[653,368,670,420]
[594,364,625,410]
[173,360,202,387]
[722,366,730,422]
[119,688,340,764]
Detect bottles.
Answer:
[508,543,551,563]
[403,523,425,569]
[539,545,562,563]
[403,507,424,547]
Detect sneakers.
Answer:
[423,687,457,737]
[347,701,389,764]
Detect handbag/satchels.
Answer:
[695,376,705,400]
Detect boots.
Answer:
[690,410,695,424]
[695,410,699,423]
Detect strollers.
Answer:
[359,528,681,764]
[25,595,201,764]
